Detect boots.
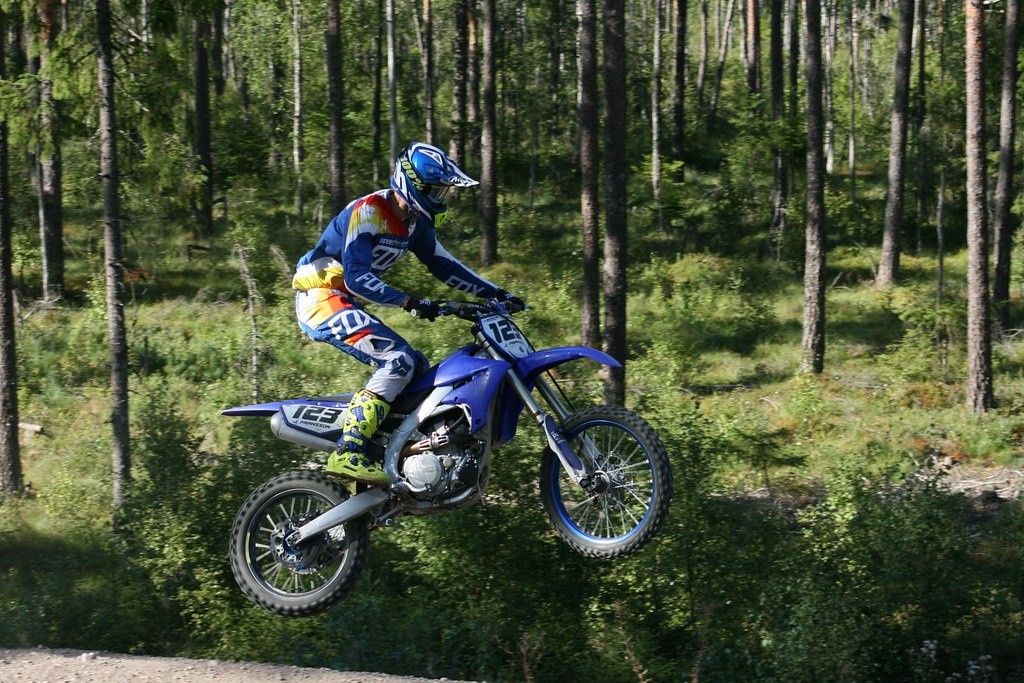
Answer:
[326,387,389,487]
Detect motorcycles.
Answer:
[218,302,675,616]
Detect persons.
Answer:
[291,146,525,483]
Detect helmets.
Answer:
[390,142,480,227]
[399,153,452,203]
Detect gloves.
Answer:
[496,289,525,313]
[404,298,442,322]
